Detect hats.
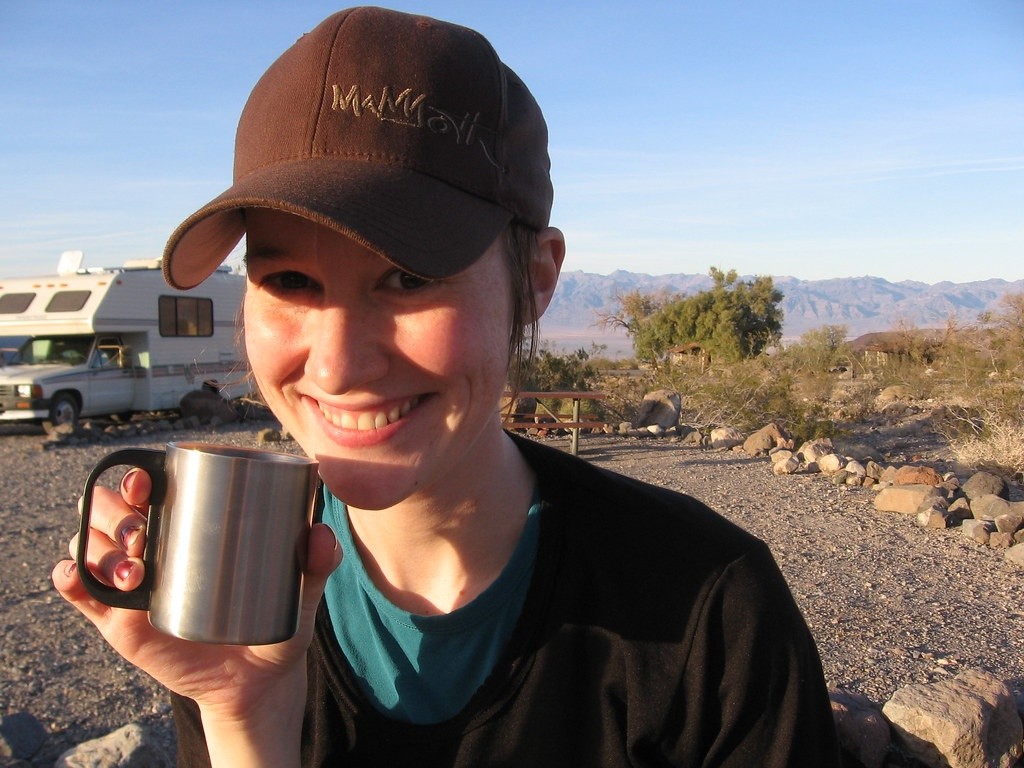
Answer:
[162,7,554,291]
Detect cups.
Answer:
[77,443,321,645]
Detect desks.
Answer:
[501,391,604,458]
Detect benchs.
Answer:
[500,414,599,418]
[502,420,604,428]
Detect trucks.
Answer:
[0,250,259,434]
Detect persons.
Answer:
[53,6,844,768]
